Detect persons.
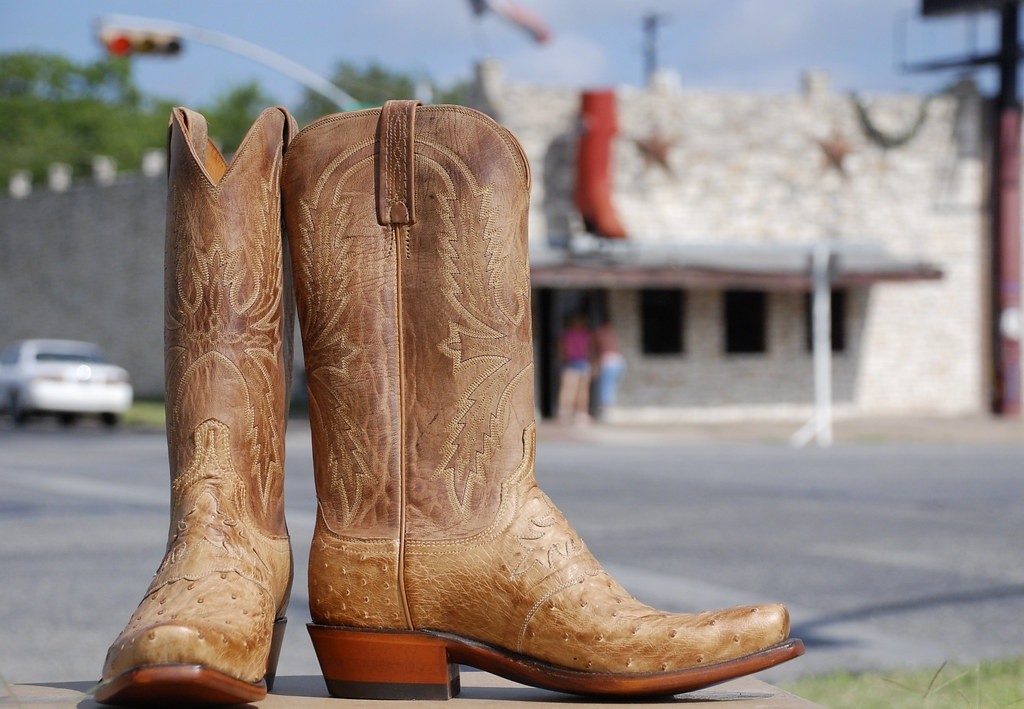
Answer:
[556,306,623,429]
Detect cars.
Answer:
[0,334,136,426]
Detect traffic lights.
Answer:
[99,25,183,59]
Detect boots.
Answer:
[284,99,805,700]
[95,105,302,702]
